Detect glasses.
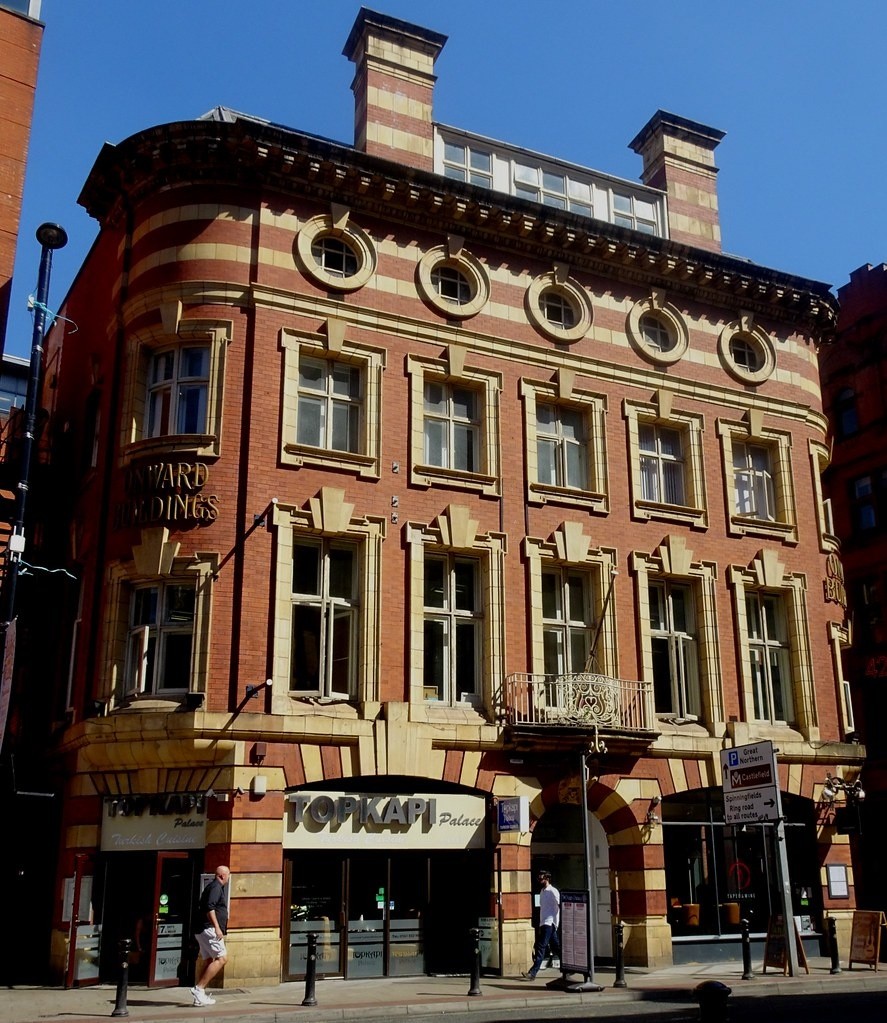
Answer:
[537,877,543,880]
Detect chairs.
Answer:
[390,909,420,958]
[315,916,353,966]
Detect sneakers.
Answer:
[189,986,215,1004]
[193,993,215,1006]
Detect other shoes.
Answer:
[522,972,535,981]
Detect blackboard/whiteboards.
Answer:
[765,916,807,968]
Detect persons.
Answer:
[128,910,152,981]
[522,870,560,981]
[190,866,230,1006]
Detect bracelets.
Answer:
[139,949,141,951]
